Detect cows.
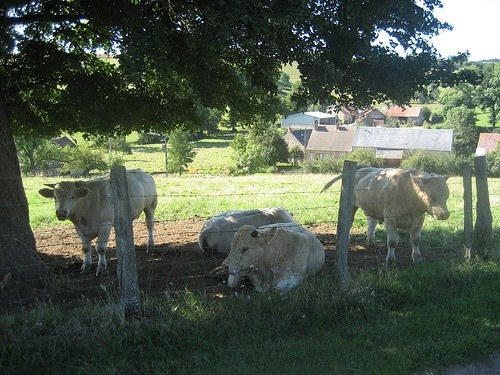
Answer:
[38,168,158,277]
[317,164,451,268]
[198,206,326,295]
